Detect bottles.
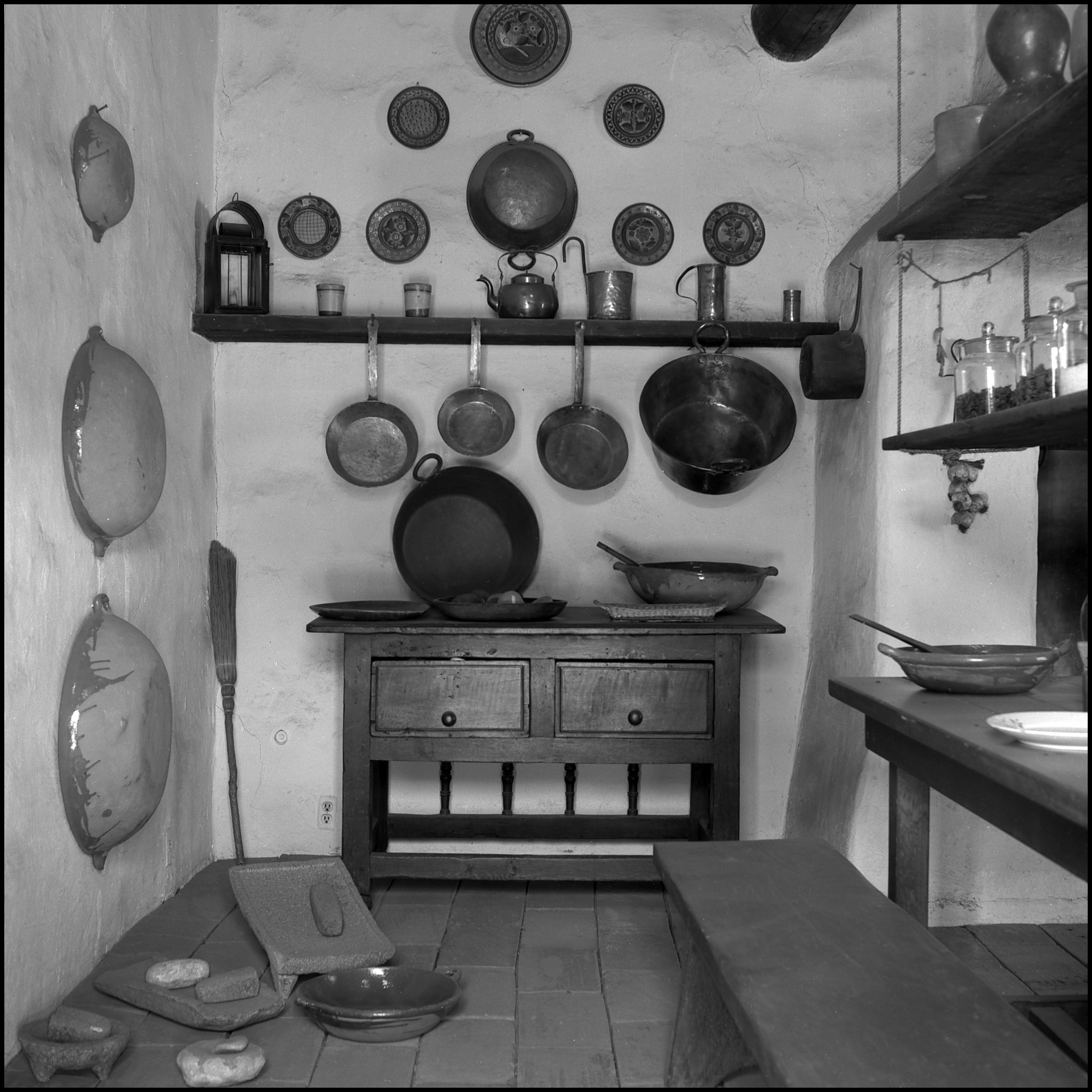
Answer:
[953,281,1088,422]
[979,5,1071,151]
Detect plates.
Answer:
[603,83,665,148]
[309,601,431,621]
[278,196,341,261]
[433,596,568,623]
[387,86,450,149]
[702,202,765,266]
[366,199,431,264]
[611,203,674,266]
[985,710,1088,752]
[469,5,572,88]
[594,599,729,623]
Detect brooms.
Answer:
[205,539,250,865]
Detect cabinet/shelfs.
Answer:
[308,602,786,911]
[880,67,1092,452]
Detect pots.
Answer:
[56,593,173,870]
[466,129,578,272]
[324,320,797,496]
[392,453,540,605]
[612,560,779,616]
[59,322,167,557]
[798,267,866,401]
[71,108,135,241]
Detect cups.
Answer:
[675,263,727,320]
[316,283,346,316]
[563,236,633,320]
[783,290,802,322]
[933,103,989,183]
[403,283,432,317]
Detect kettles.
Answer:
[476,250,559,320]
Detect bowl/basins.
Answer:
[877,640,1073,695]
[293,965,462,1043]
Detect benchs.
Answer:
[654,835,1088,1088]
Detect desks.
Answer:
[829,673,1089,931]
